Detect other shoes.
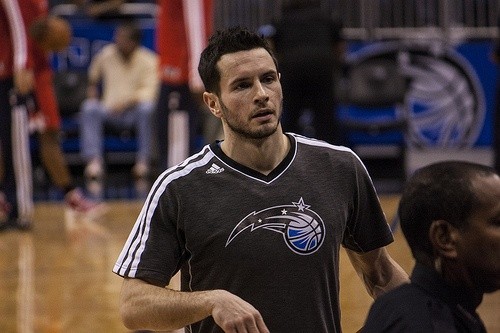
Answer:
[62,188,110,222]
[82,164,102,180]
[132,162,148,176]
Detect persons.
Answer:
[272,0,352,151]
[77,22,160,177]
[155,0,216,181]
[0,0,110,222]
[356,159,500,333]
[112,23,411,333]
